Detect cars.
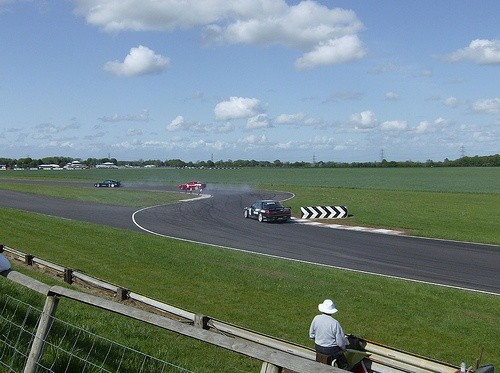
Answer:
[94,179,120,187]
[179,181,206,192]
[244,200,291,223]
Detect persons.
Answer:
[0,245,11,273]
[307,299,349,368]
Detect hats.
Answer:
[318,300,338,314]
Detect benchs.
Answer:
[344,333,475,373]
